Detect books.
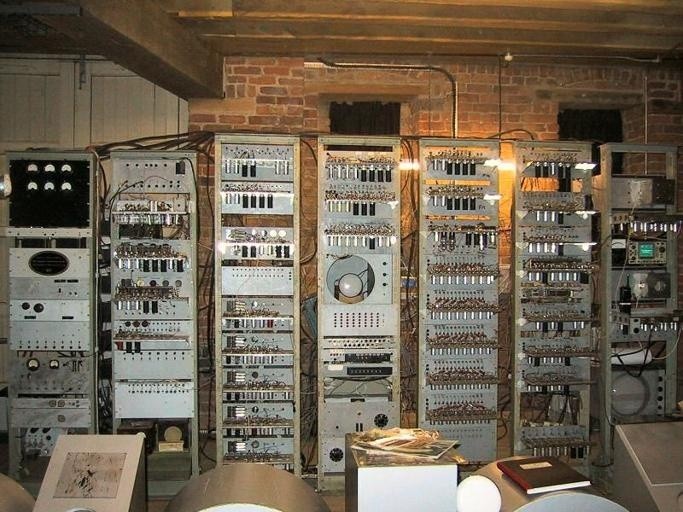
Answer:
[350,428,459,466]
[495,455,592,495]
